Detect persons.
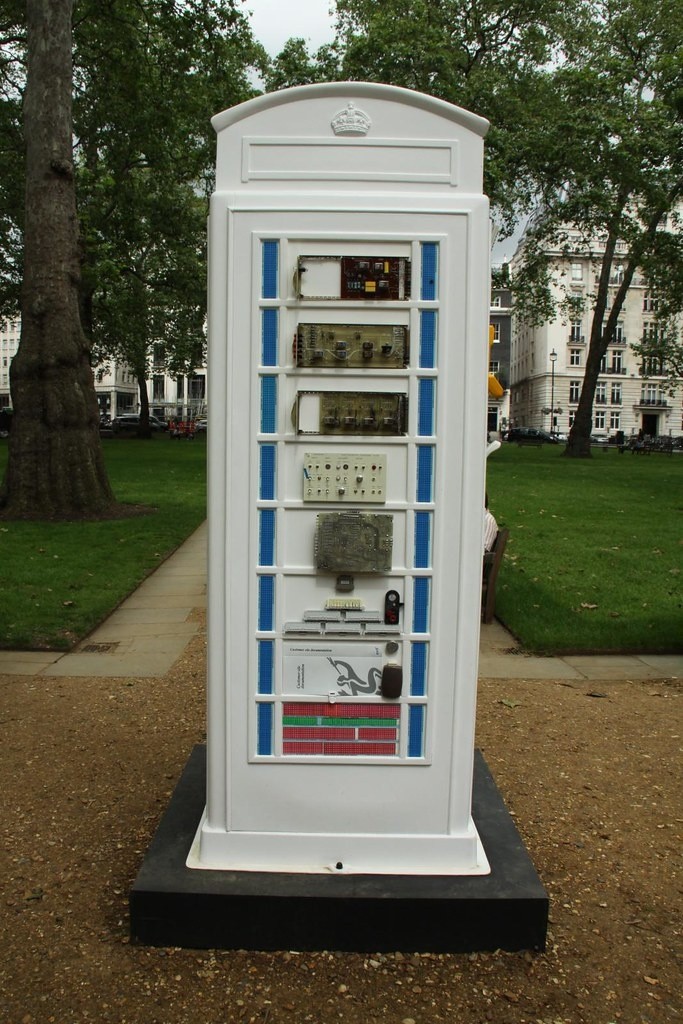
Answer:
[483,492,499,555]
[170,413,182,435]
[639,429,644,437]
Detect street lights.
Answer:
[550,348,557,433]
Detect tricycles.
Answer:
[170,404,198,439]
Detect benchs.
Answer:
[618,435,683,457]
[518,438,544,448]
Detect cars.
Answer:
[110,413,168,431]
[550,431,610,443]
[507,428,557,442]
